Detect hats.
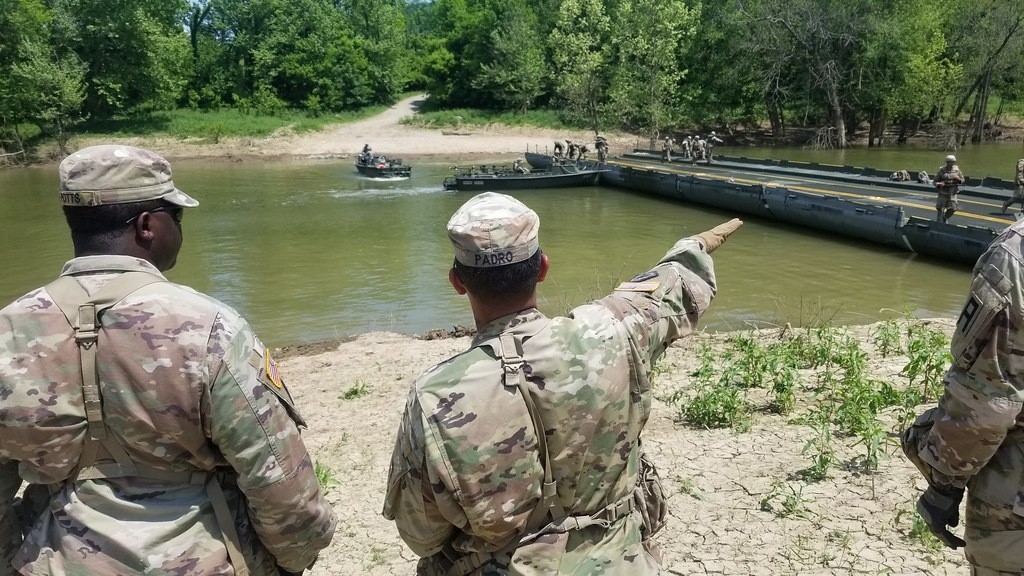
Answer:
[58,145,199,208]
[445,192,540,268]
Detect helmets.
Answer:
[711,131,716,135]
[688,136,692,139]
[665,136,669,140]
[945,155,956,163]
[695,135,699,139]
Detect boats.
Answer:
[441,142,613,193]
[353,151,414,180]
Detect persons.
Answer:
[661,136,676,164]
[378,156,385,164]
[899,217,1024,576]
[933,155,965,224]
[1002,159,1024,213]
[682,131,723,166]
[574,144,590,161]
[363,144,373,164]
[553,141,565,155]
[565,139,577,159]
[595,135,609,162]
[513,158,525,172]
[0,145,339,576]
[380,192,744,576]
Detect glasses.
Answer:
[125,207,183,223]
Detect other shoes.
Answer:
[943,217,948,224]
[1001,202,1007,214]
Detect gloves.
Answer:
[695,217,743,253]
[917,485,967,550]
